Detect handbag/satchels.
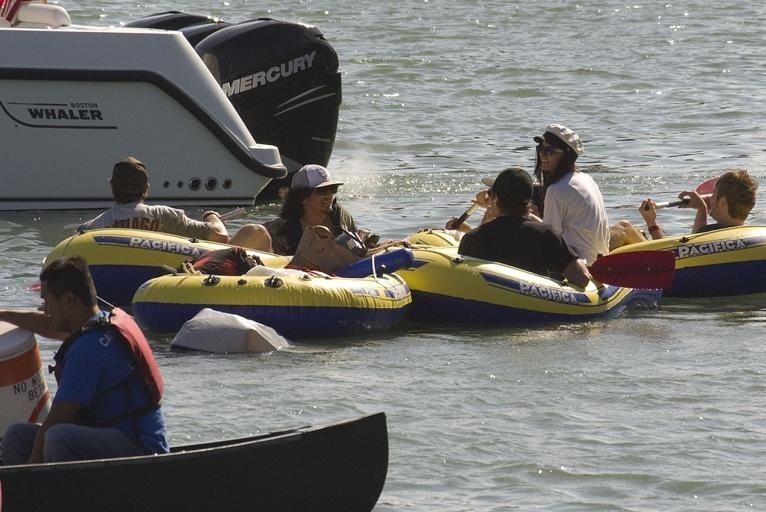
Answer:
[284,225,364,270]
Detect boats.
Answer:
[587,223,766,300]
[391,226,666,320]
[0,410,389,512]
[131,255,412,337]
[0,0,342,210]
[33,226,296,308]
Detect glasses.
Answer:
[319,188,337,193]
[536,146,566,155]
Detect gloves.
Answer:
[202,210,221,221]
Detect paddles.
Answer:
[645,177,717,208]
[585,249,677,292]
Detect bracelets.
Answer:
[203,212,216,220]
[648,225,661,234]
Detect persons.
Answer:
[76,157,276,251]
[618,171,758,245]
[458,167,587,285]
[0,255,167,465]
[531,123,610,270]
[446,183,543,232]
[230,164,379,252]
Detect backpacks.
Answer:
[179,247,264,276]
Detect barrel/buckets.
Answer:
[0,318,52,433]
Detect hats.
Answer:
[534,123,584,161]
[107,157,149,195]
[291,165,344,189]
[481,168,534,202]
